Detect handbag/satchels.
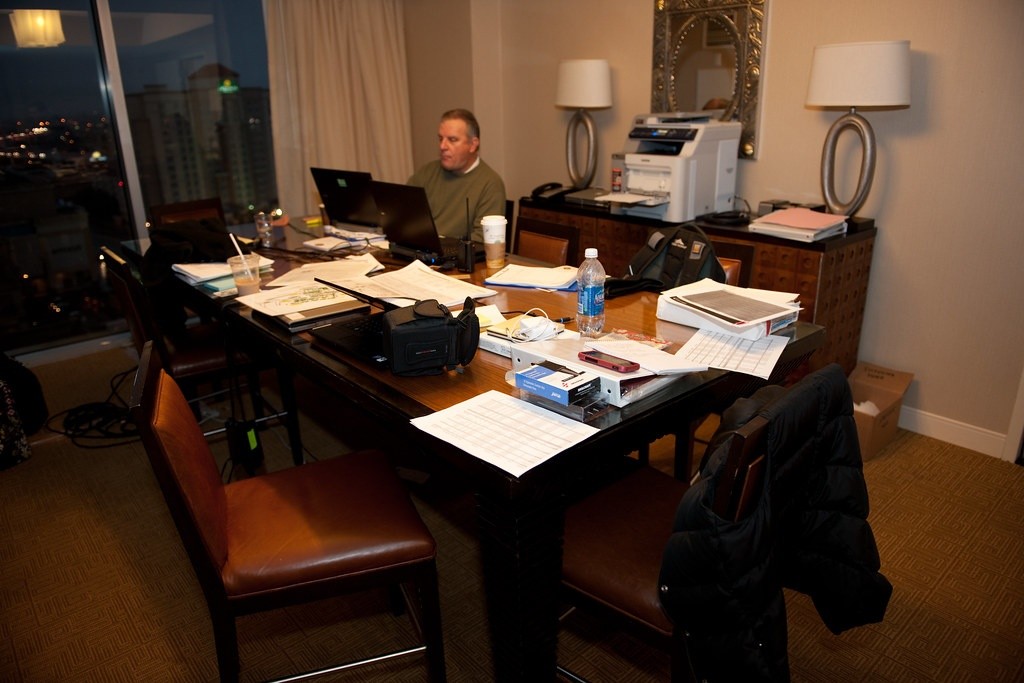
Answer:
[384,297,480,376]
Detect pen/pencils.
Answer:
[524,396,608,419]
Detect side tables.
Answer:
[507,203,877,385]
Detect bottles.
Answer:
[611,154,625,194]
[577,248,606,336]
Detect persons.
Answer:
[406,108,505,243]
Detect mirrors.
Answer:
[653,0,767,161]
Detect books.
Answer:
[276,299,371,333]
[171,251,275,299]
[304,225,386,252]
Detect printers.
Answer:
[594,110,742,222]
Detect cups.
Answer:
[481,215,507,268]
[319,205,330,225]
[257,215,273,246]
[228,255,260,297]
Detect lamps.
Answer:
[8,10,66,50]
[804,40,911,223]
[553,57,615,192]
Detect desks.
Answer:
[116,219,827,683]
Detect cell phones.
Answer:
[578,351,640,373]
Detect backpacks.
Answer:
[626,222,726,287]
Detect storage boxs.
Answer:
[847,357,913,463]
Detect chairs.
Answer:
[508,216,581,267]
[563,363,839,682]
[139,364,447,683]
[711,241,755,290]
[95,245,304,476]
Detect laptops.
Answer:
[310,167,487,265]
[307,277,403,370]
